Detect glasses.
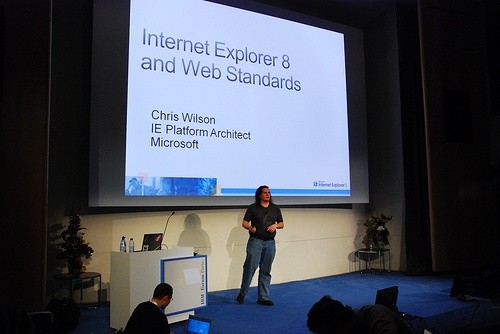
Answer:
[168,296,173,300]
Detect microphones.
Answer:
[158,212,175,250]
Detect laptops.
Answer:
[185,314,212,334]
[134,232,163,252]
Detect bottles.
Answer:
[129,238,134,252]
[120,236,126,252]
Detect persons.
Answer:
[122,283,173,334]
[236,186,284,306]
[307,296,413,334]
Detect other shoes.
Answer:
[237,293,245,304]
[257,299,274,306]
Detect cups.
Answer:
[370,244,372,250]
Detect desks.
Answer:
[53,272,102,310]
[358,248,391,273]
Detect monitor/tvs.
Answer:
[375,286,398,312]
[450,267,496,302]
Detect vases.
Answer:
[376,241,384,250]
[68,255,83,275]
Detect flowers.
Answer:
[53,215,94,261]
[364,214,393,245]
